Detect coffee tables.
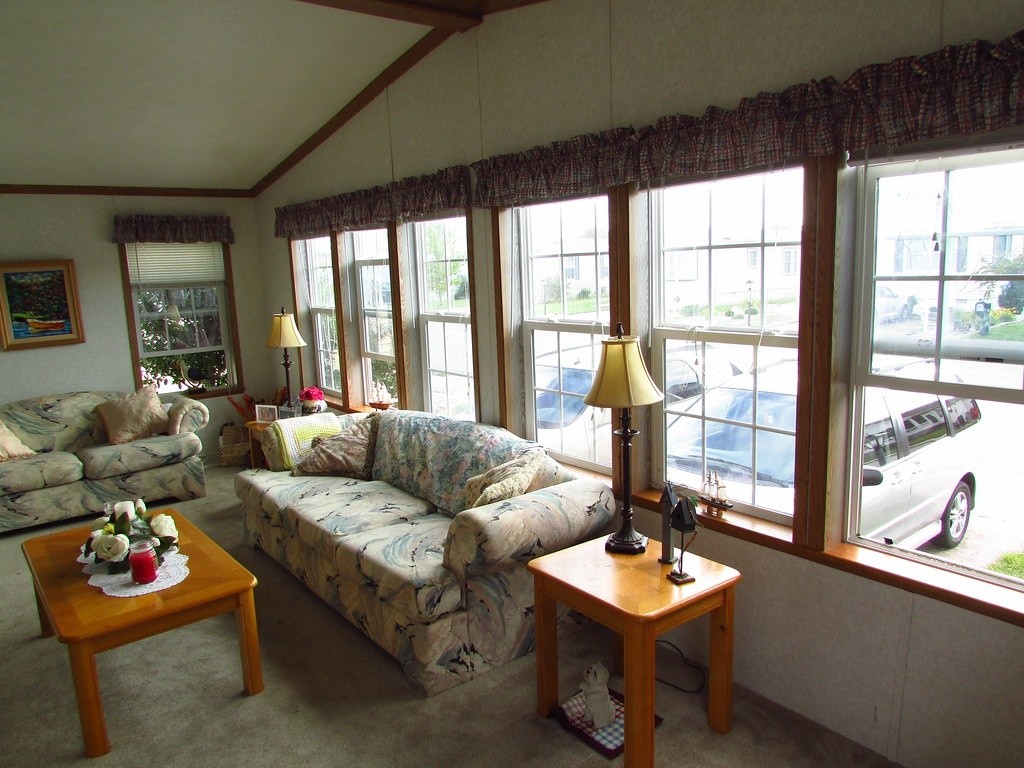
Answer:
[21,508,265,759]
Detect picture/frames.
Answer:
[0,258,86,353]
[255,405,278,423]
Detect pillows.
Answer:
[463,448,545,509]
[0,419,40,463]
[291,410,383,481]
[94,383,170,445]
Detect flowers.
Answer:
[84,497,179,575]
[299,386,325,401]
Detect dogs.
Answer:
[580,661,616,730]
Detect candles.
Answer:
[114,501,135,521]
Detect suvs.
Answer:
[665,363,986,551]
[533,348,724,459]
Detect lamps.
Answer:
[582,321,666,556]
[263,307,307,408]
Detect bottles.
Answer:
[129,540,159,584]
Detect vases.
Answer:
[304,400,328,412]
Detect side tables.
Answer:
[527,531,742,768]
[244,421,271,468]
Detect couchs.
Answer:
[234,410,617,699]
[0,391,210,536]
